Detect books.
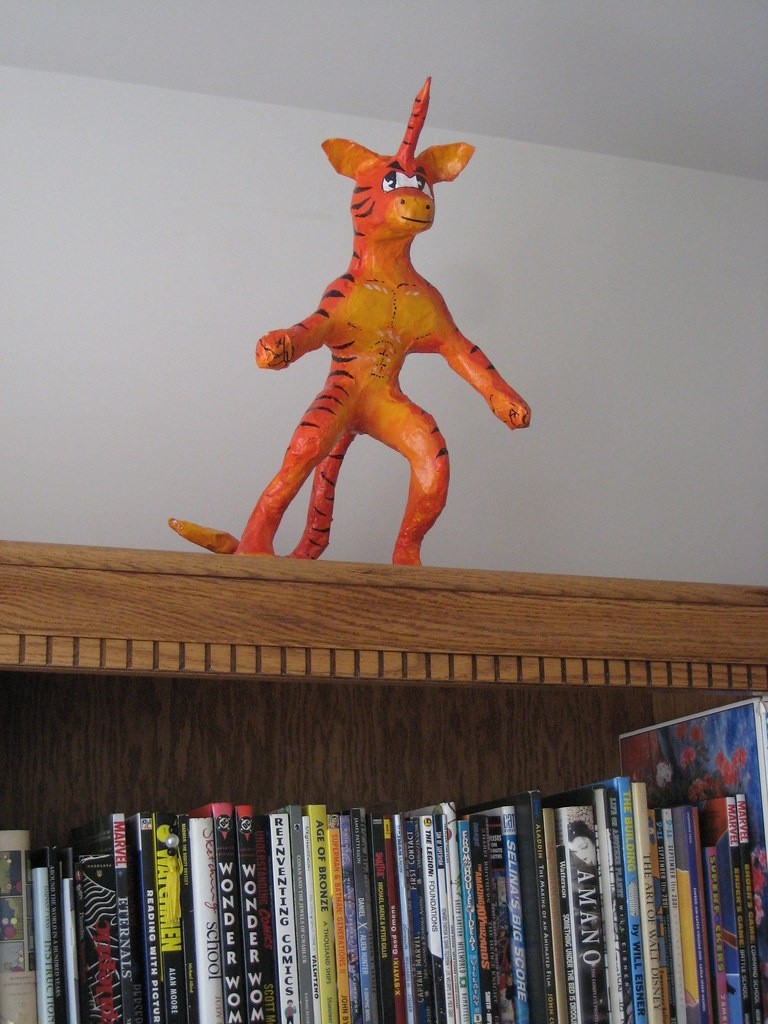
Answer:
[1,696,768,1024]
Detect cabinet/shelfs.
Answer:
[0,538,768,847]
